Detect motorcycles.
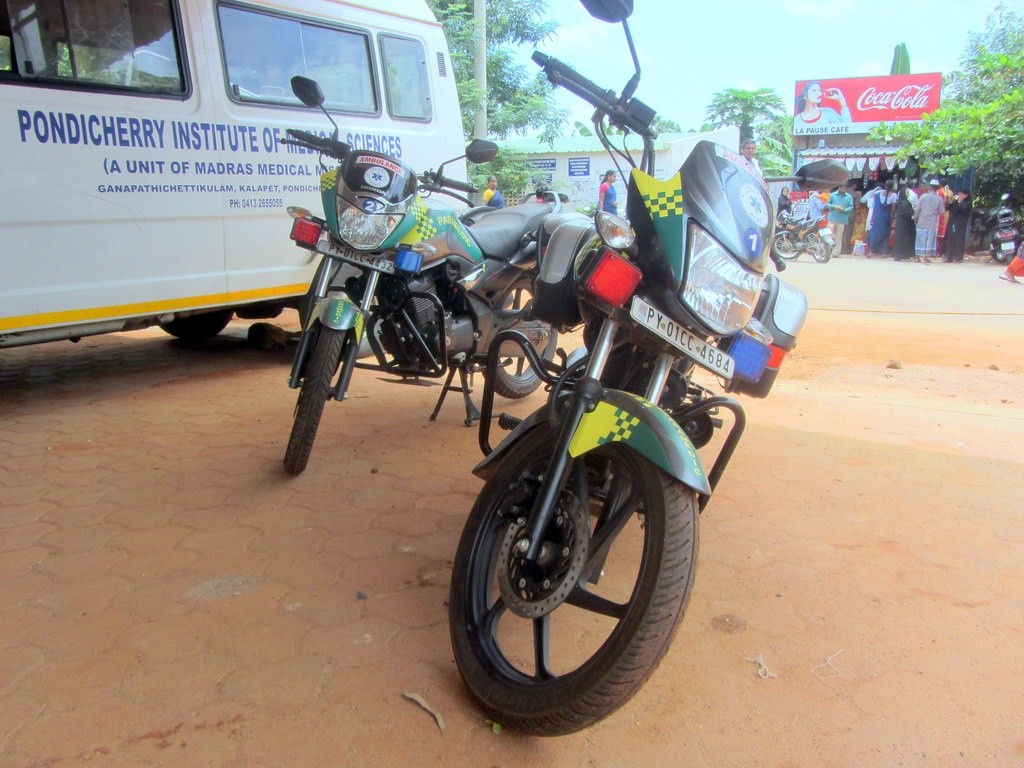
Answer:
[283,75,569,472]
[447,0,853,737]
[772,201,836,263]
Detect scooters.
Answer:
[976,192,1022,266]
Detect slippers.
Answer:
[999,275,1019,283]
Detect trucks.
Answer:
[0,1,472,346]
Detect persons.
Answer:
[482,175,504,209]
[771,176,972,263]
[597,170,618,216]
[999,241,1024,284]
[740,140,762,177]
[794,81,852,126]
